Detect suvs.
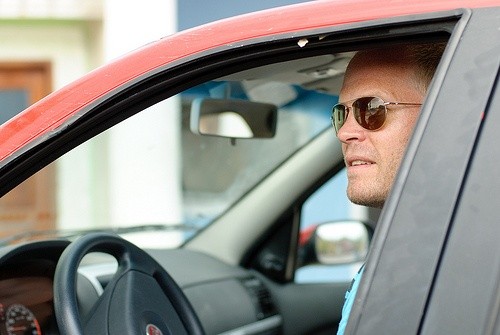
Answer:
[0,1,499,335]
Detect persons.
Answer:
[330,36,452,334]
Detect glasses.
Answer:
[331,96,424,133]
